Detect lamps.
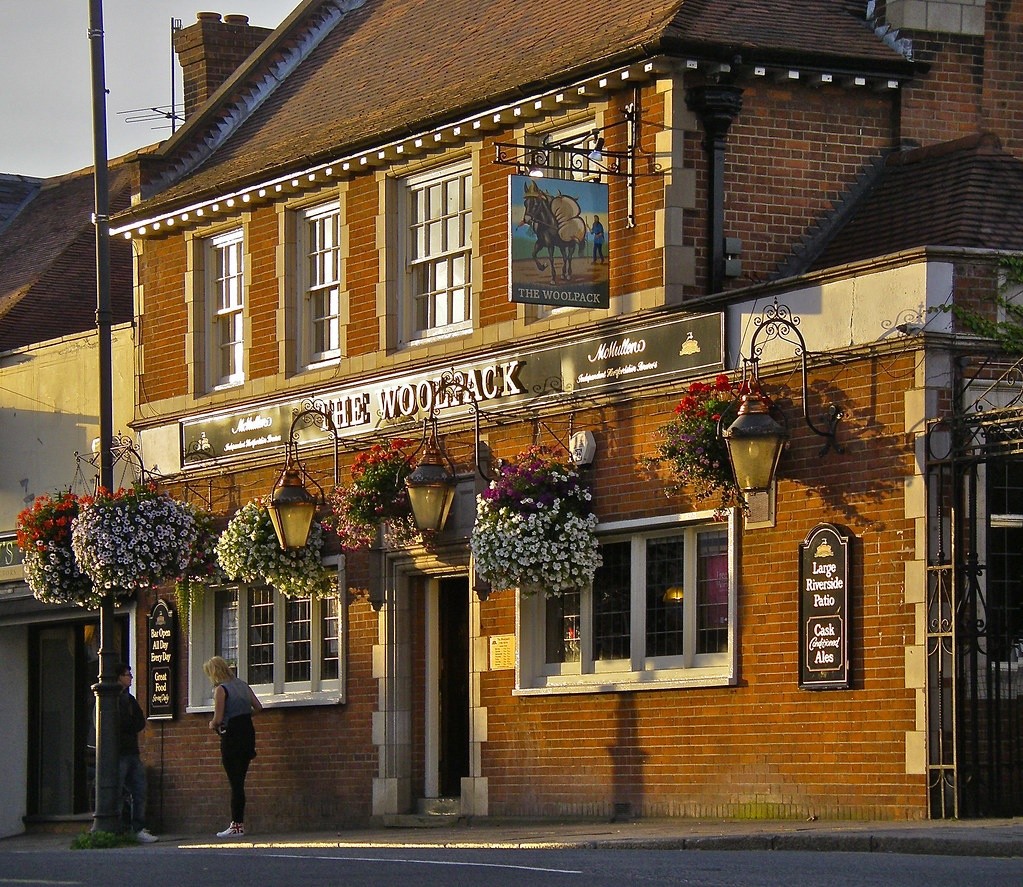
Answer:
[395,365,498,533]
[588,130,605,162]
[265,395,338,553]
[661,582,683,603]
[715,295,848,491]
[529,169,546,178]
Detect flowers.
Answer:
[18,438,417,610]
[633,374,773,523]
[470,444,605,599]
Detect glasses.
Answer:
[120,674,132,678]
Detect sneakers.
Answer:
[217,820,245,838]
[133,828,159,843]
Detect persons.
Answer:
[89,662,161,844]
[202,656,264,837]
